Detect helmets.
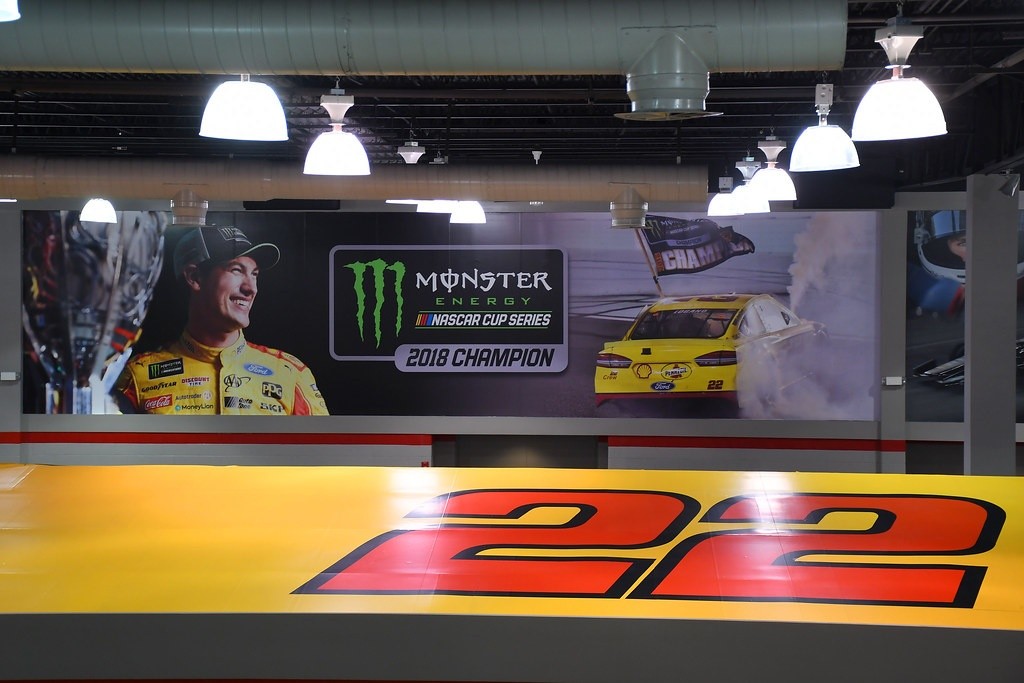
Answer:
[915,210,1024,286]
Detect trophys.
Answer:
[19,208,167,415]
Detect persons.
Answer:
[905,210,1023,324]
[116,224,330,415]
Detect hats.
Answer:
[173,225,280,280]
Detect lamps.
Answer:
[708,127,797,216]
[198,74,289,142]
[789,71,861,172]
[851,1,948,141]
[79,197,117,223]
[302,77,371,177]
[386,128,486,224]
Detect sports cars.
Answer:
[594,291,830,410]
